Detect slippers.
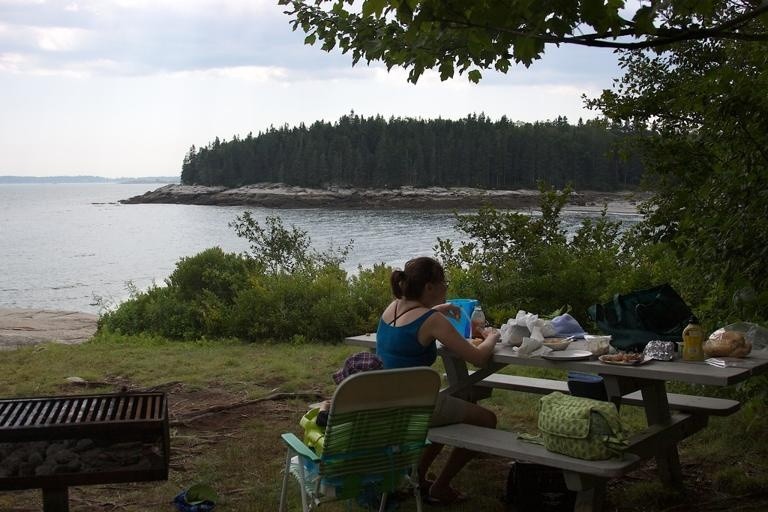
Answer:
[422,488,472,507]
[408,479,434,495]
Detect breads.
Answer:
[704,332,752,357]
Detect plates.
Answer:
[598,353,653,365]
[540,350,593,360]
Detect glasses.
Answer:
[433,278,451,287]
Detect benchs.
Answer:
[443,370,740,417]
[309,401,642,478]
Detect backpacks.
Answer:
[499,461,579,512]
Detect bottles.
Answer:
[680,316,705,363]
[470,306,487,341]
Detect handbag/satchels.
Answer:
[585,279,701,354]
[515,392,632,460]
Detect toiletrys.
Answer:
[682,318,703,360]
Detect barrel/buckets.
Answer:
[444,298,479,339]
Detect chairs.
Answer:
[278,366,441,512]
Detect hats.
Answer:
[331,350,386,385]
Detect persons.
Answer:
[373,255,502,500]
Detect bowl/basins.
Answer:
[542,337,575,351]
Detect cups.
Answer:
[584,335,610,360]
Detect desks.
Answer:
[345,334,767,511]
